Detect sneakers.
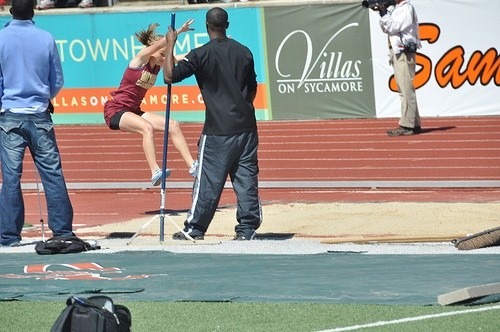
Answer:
[36,0,55,8]
[78,0,93,7]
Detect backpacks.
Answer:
[50,295,132,331]
[35,235,101,255]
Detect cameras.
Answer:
[362,0,394,8]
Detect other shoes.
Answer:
[173,228,204,241]
[189,159,198,176]
[151,169,171,186]
[387,126,414,135]
[414,126,423,133]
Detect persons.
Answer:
[370,0,423,136]
[163,7,263,241]
[0,0,76,247]
[103,18,198,187]
